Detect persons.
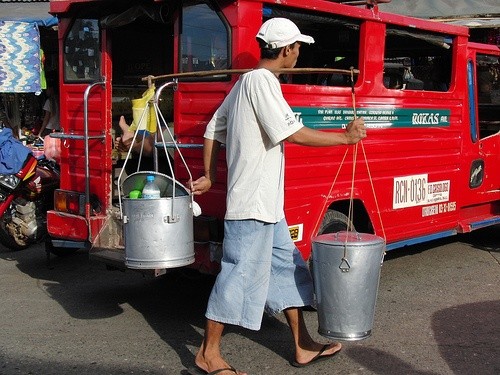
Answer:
[185,18,367,375]
[37,75,60,141]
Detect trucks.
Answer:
[46,0,500,274]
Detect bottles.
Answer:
[141,174,160,199]
[129,191,141,199]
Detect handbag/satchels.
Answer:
[44,129,61,164]
[127,83,157,134]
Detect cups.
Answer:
[132,109,148,134]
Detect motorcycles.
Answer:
[0,120,61,251]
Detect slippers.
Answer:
[187,363,238,375]
[291,342,342,368]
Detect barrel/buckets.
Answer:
[311,230,386,343]
[119,171,196,270]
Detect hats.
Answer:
[255,17,315,49]
